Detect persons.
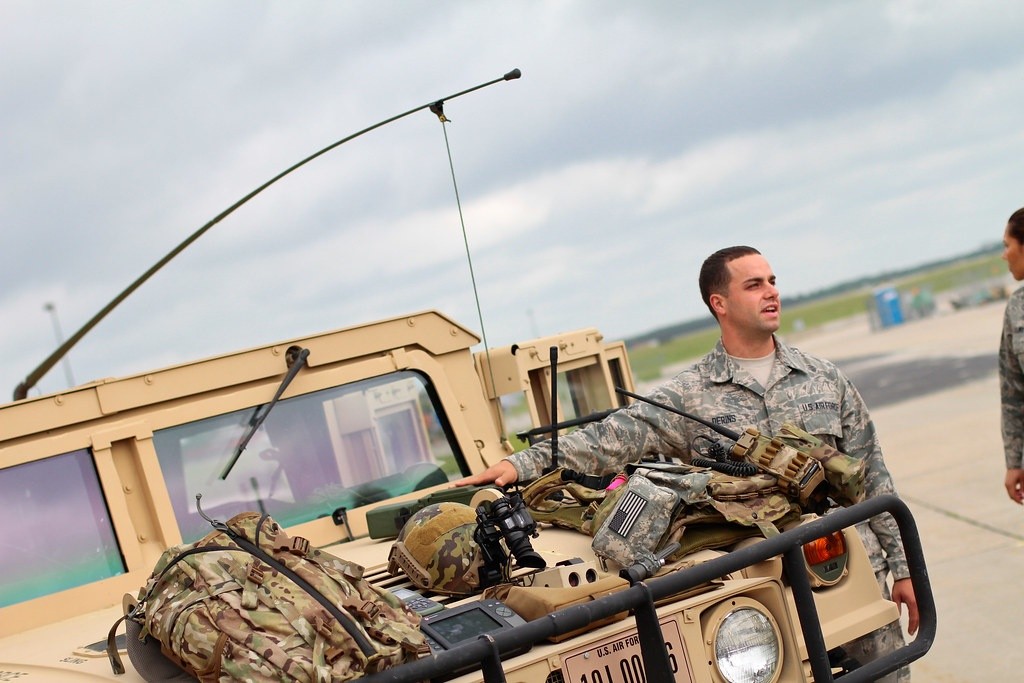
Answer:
[999,209,1024,503]
[455,246,921,683]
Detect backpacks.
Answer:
[106,510,439,682]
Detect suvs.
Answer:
[0,308,937,683]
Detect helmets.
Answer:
[388,501,512,593]
